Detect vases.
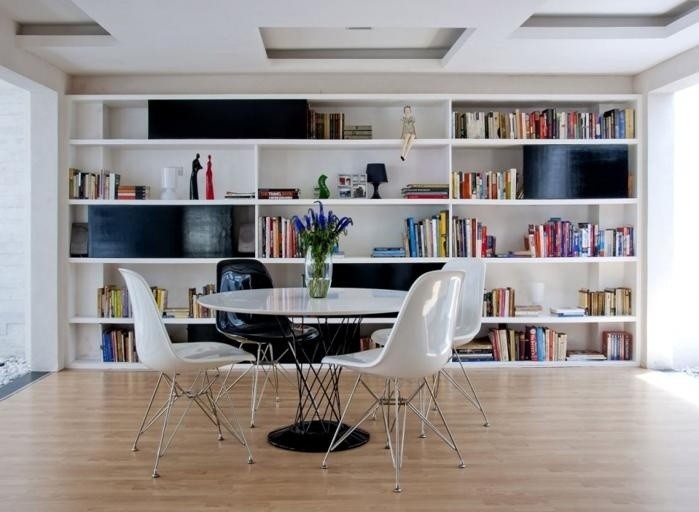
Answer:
[304,246,333,298]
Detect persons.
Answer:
[399,105,416,162]
[206,154,214,200]
[189,152,202,199]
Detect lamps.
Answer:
[365,163,388,199]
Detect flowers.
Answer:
[289,201,353,296]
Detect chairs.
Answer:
[119,267,258,477]
[321,270,466,492]
[371,258,489,438]
[210,256,319,429]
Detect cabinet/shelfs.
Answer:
[64,93,644,371]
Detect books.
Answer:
[452,216,634,258]
[371,210,449,257]
[103,329,139,363]
[69,168,151,200]
[452,324,631,362]
[452,108,636,138]
[481,287,543,317]
[309,109,373,139]
[259,216,307,257]
[549,287,633,317]
[258,188,299,199]
[97,284,215,319]
[451,168,525,200]
[360,337,385,353]
[401,183,449,199]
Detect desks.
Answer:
[197,288,410,452]
